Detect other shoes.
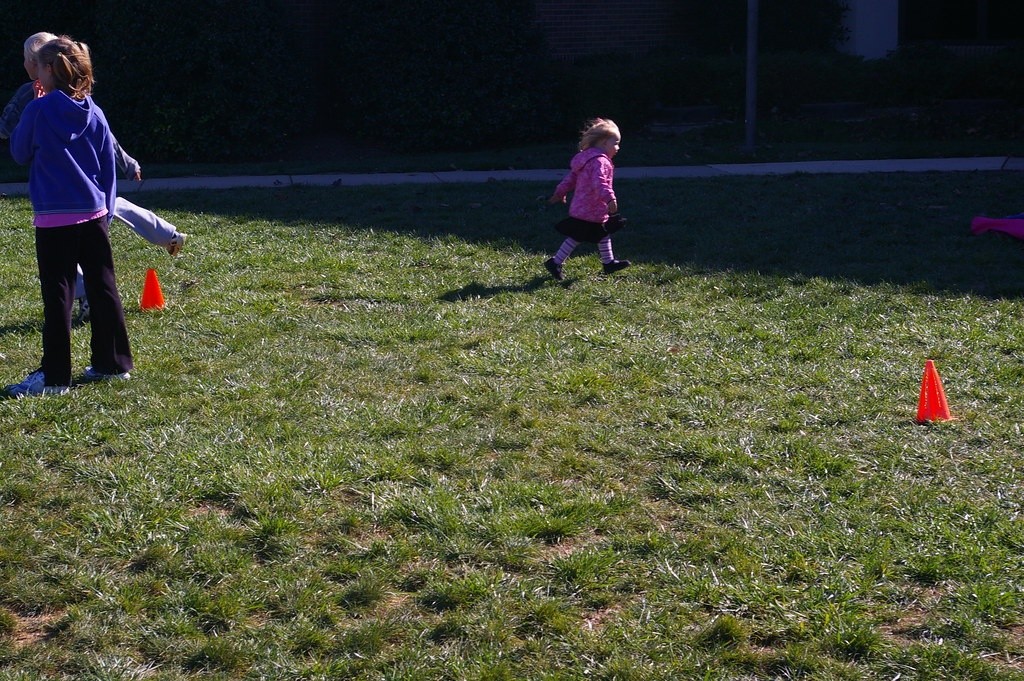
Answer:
[167,231,186,255]
[78,301,91,322]
[544,258,565,281]
[603,260,630,274]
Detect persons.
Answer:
[3,38,138,397]
[545,119,631,280]
[0,32,189,324]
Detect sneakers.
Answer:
[7,371,69,398]
[84,365,130,381]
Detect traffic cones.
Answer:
[917,360,951,422]
[140,266,166,310]
